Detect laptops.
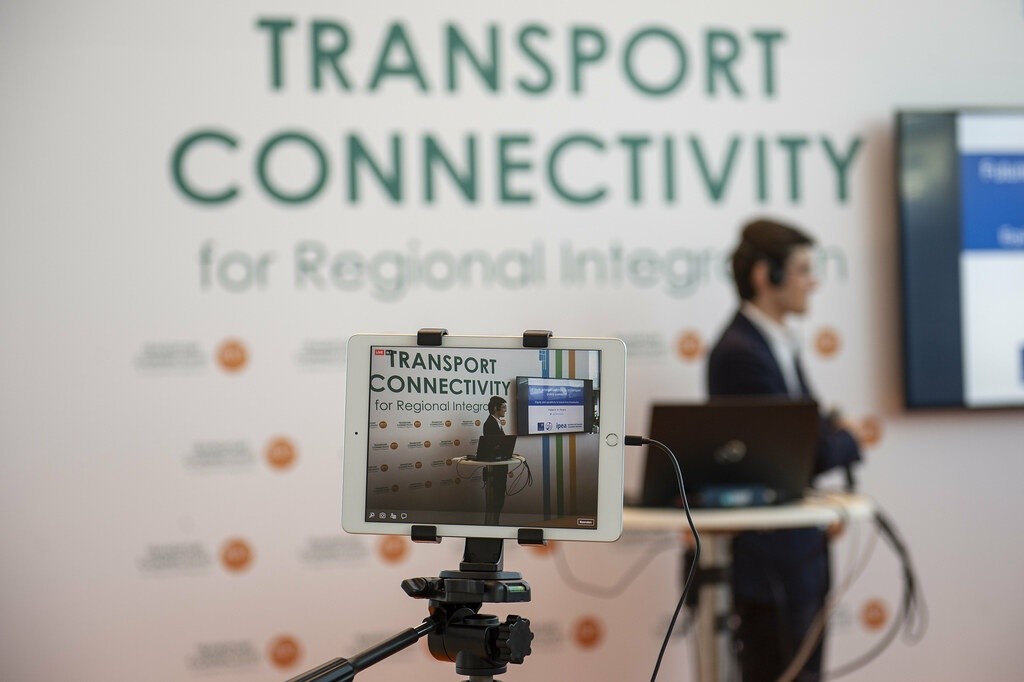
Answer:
[623,399,820,511]
[464,435,518,462]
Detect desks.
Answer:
[622,489,876,682]
[451,456,526,526]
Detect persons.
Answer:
[484,396,508,526]
[708,218,881,682]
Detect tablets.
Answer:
[339,332,627,542]
